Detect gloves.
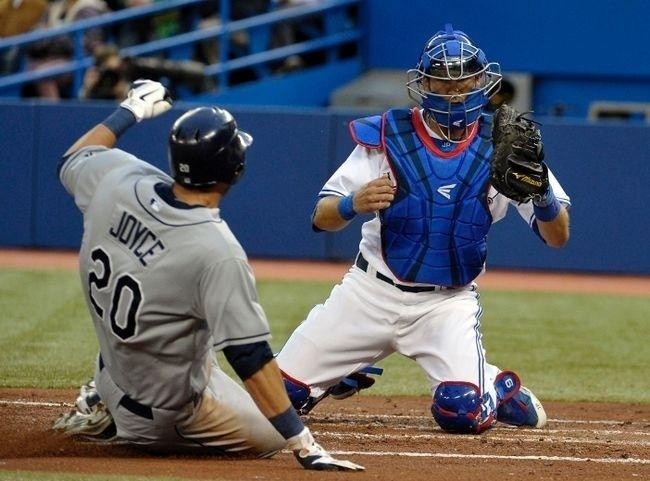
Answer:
[117,74,174,125]
[284,425,367,472]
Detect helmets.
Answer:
[167,105,257,188]
[404,21,507,145]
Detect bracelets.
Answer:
[531,183,561,222]
[337,192,357,222]
[268,402,305,439]
[101,105,137,141]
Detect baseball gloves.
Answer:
[489,104,555,207]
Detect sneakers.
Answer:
[74,378,108,415]
[52,400,118,443]
[492,382,549,429]
[330,369,376,401]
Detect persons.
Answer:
[1,1,356,102]
[273,22,572,435]
[51,78,366,472]
